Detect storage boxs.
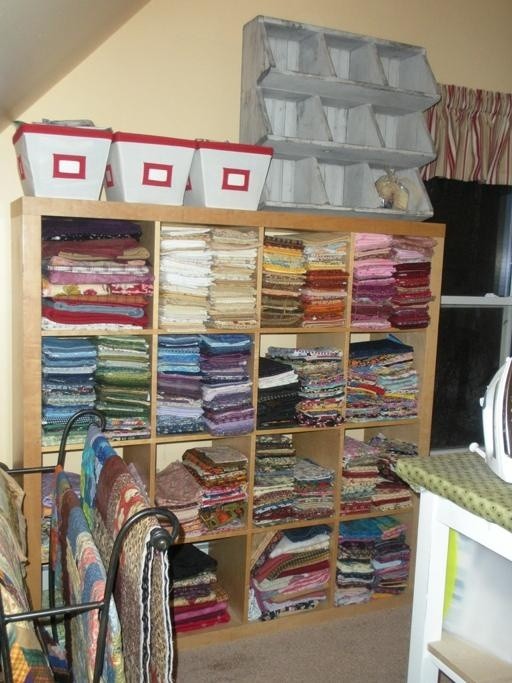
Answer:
[9,120,277,213]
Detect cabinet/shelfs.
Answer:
[3,195,451,683]
[390,485,511,683]
[241,13,443,223]
